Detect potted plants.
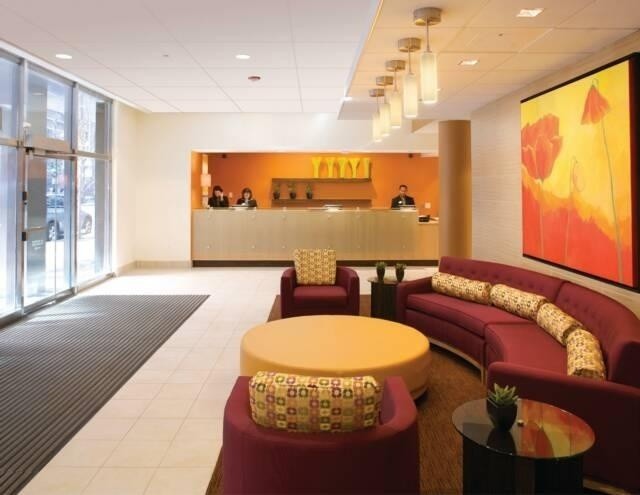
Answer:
[376,261,387,279]
[487,382,519,432]
[395,263,407,282]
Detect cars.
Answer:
[19,192,94,243]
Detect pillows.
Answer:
[431,272,491,305]
[566,328,606,380]
[293,248,336,285]
[490,284,550,321]
[536,303,587,346]
[248,370,384,433]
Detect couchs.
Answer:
[223,375,420,494]
[486,282,639,493]
[281,266,360,318]
[398,256,570,385]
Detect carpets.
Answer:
[1,294,209,494]
[205,293,622,495]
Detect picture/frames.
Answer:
[520,55,640,289]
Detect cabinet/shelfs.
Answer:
[270,177,377,210]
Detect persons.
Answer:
[236,188,258,207]
[208,185,230,207]
[390,184,416,209]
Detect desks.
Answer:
[368,278,397,320]
[452,397,596,493]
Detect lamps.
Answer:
[376,76,393,137]
[370,89,384,143]
[385,59,405,128]
[399,38,420,118]
[413,7,441,104]
